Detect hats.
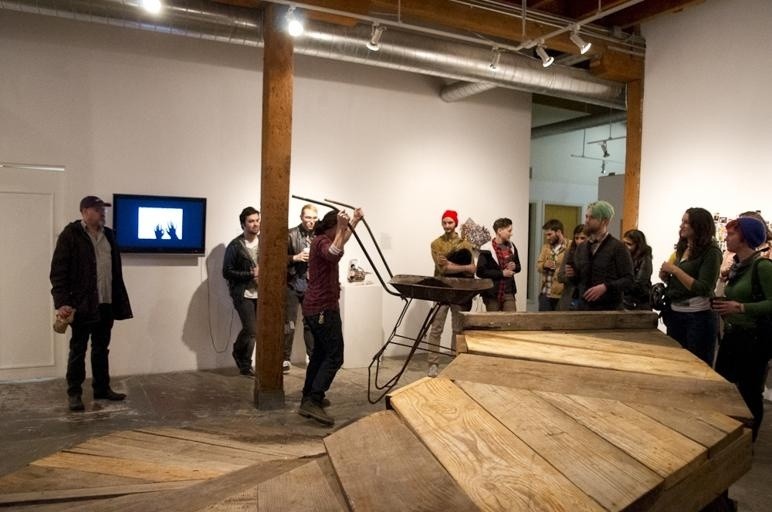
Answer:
[80,196,111,208]
[739,217,766,247]
[442,210,458,225]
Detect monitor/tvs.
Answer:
[112,193,207,258]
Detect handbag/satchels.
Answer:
[651,283,668,310]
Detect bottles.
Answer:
[54,305,71,334]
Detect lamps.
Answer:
[600,160,608,174]
[284,4,386,53]
[487,24,596,72]
[599,141,611,159]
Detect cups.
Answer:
[546,255,555,268]
[709,296,726,310]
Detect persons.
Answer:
[621,230,652,311]
[50,197,133,410]
[223,207,259,377]
[558,224,592,312]
[428,210,476,377]
[569,200,633,311]
[299,207,363,425]
[282,205,318,370]
[658,208,772,442]
[477,218,520,312]
[538,220,572,312]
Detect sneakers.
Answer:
[282,361,291,374]
[298,396,335,425]
[428,364,438,377]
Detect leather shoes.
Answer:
[68,396,85,409]
[94,390,126,401]
